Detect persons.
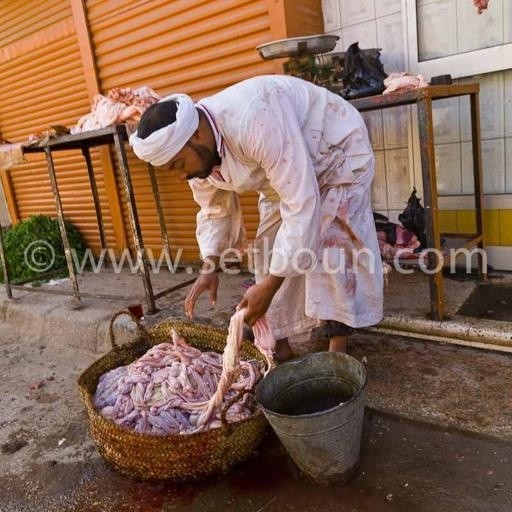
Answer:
[129,75,384,350]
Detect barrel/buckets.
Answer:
[254,351,369,487]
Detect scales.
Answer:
[255,34,383,94]
